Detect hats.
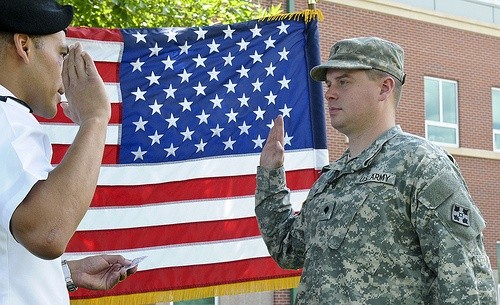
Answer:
[310,37,406,85]
[0,0,74,36]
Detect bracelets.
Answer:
[61,259,78,291]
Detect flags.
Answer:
[31,10,329,305]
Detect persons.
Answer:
[255,37,496,305]
[0,0,137,305]
